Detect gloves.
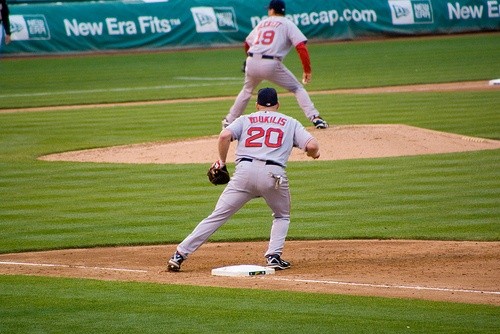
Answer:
[209,160,224,174]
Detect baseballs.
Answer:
[302,79,307,85]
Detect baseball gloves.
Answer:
[206,159,230,185]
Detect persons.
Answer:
[168,87,321,270]
[221,0,329,132]
[0,0,12,48]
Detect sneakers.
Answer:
[167,251,184,269]
[265,255,291,272]
[220,120,230,129]
[312,119,329,129]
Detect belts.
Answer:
[247,53,275,59]
[239,157,281,165]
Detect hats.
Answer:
[257,87,278,107]
[265,0,286,15]
